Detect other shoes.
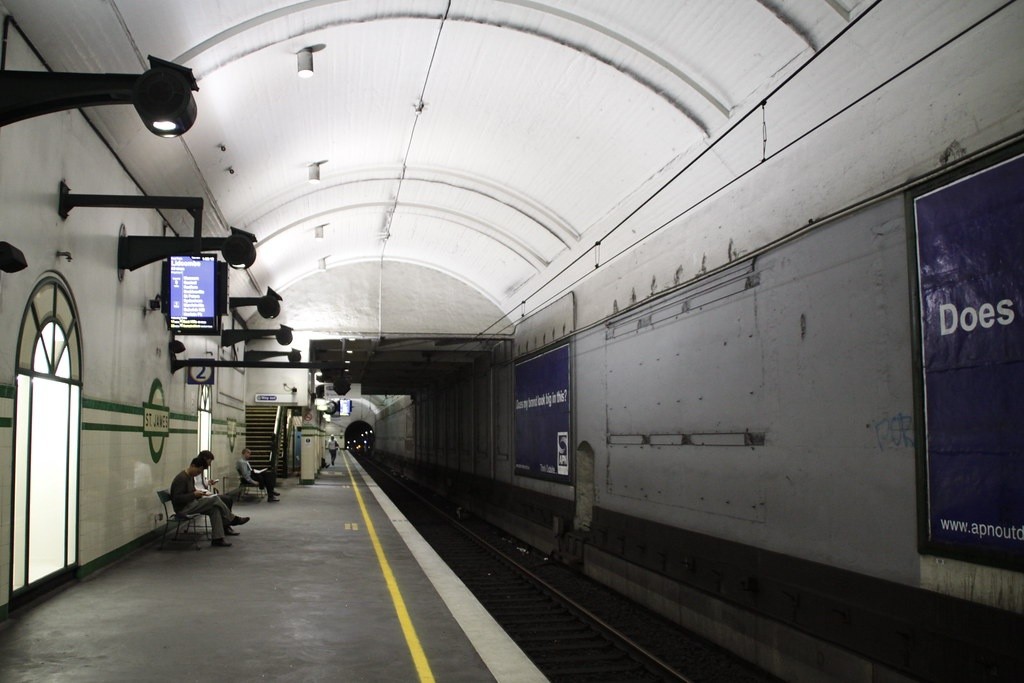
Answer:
[211,539,232,547]
[224,527,240,536]
[268,497,280,502]
[273,491,280,496]
[231,516,250,526]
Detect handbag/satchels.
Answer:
[335,441,339,448]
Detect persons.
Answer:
[170,450,250,547]
[325,434,340,466]
[235,448,280,503]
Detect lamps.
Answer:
[308,165,321,184]
[314,226,323,239]
[297,47,314,78]
[318,258,326,271]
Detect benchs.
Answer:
[235,467,267,503]
[157,488,212,551]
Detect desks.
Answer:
[223,472,249,496]
